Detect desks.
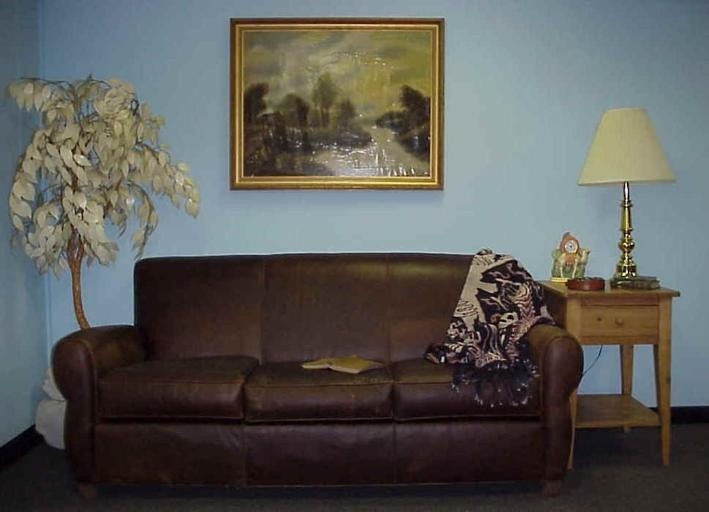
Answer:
[537,279,681,471]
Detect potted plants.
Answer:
[5,74,202,450]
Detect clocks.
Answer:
[550,231,591,283]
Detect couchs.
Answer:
[52,253,586,500]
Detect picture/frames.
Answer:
[229,17,445,191]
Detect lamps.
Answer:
[577,106,676,284]
